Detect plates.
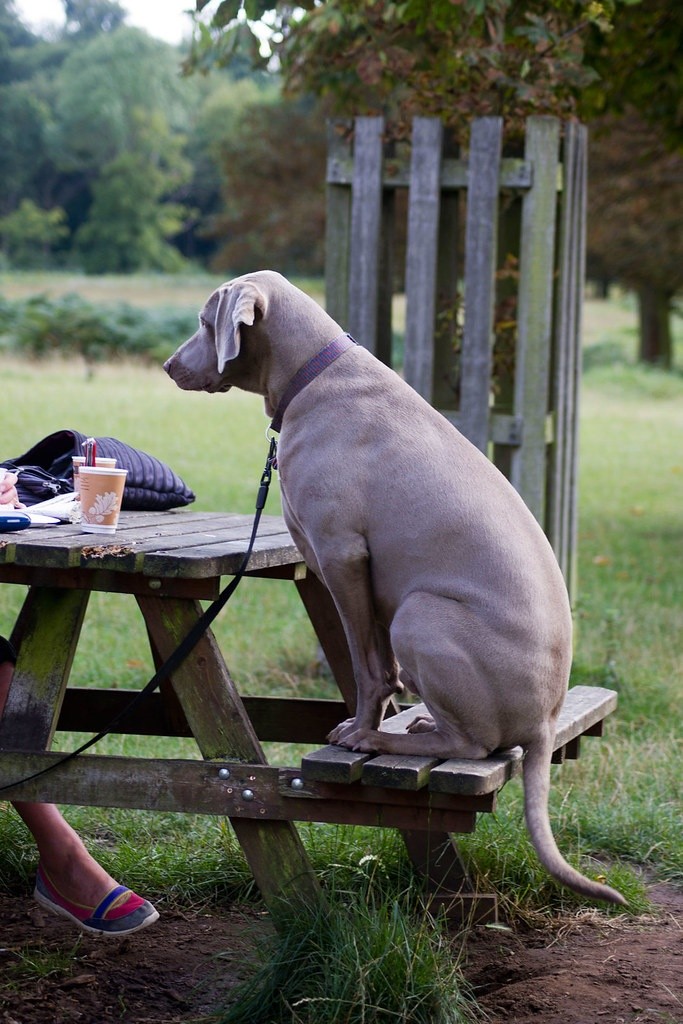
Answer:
[26,513,60,528]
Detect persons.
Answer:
[0,468,160,937]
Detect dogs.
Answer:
[163,270,631,908]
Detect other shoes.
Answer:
[34,858,158,937]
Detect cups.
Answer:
[79,466,127,535]
[72,455,116,501]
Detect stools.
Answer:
[300,667,621,800]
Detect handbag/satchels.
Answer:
[0,427,194,512]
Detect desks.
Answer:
[0,505,497,964]
